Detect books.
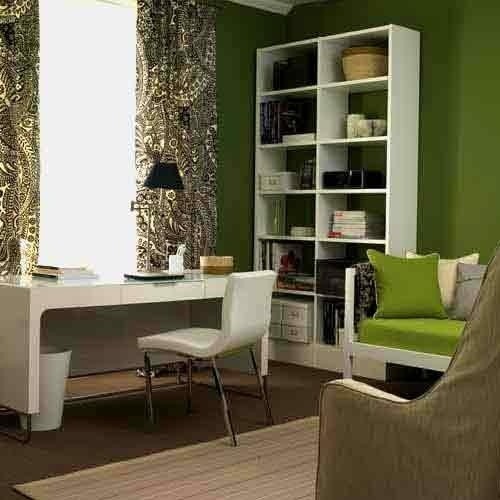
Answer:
[260,103,269,144]
[259,240,313,291]
[267,100,276,143]
[282,133,316,144]
[277,101,308,143]
[323,206,383,345]
[31,264,102,279]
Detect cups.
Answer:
[345,112,386,138]
[168,254,184,275]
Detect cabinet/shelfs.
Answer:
[252,22,420,381]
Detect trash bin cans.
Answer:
[18,345,72,432]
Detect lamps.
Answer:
[123,162,184,280]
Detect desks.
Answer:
[0,267,267,444]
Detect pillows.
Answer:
[365,247,488,320]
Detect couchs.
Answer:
[342,260,467,381]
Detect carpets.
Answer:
[13,414,318,499]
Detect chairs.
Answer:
[316,242,499,500]
[137,268,279,446]
